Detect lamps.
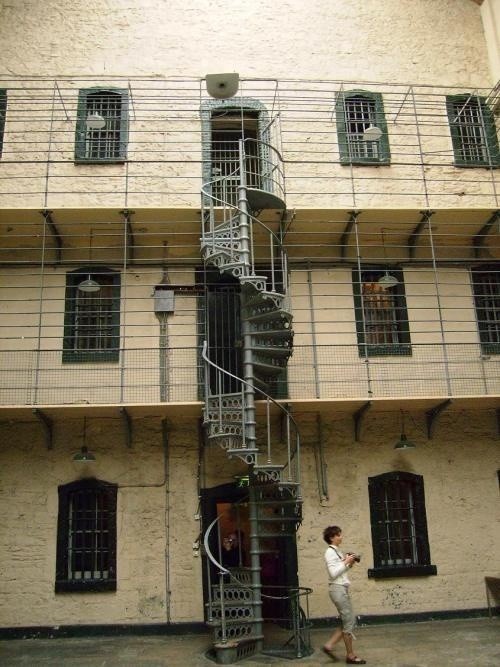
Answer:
[85,99,105,131]
[73,415,95,461]
[77,227,101,292]
[394,410,416,448]
[377,227,400,286]
[361,101,383,141]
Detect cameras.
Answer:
[349,552,360,563]
[228,539,232,542]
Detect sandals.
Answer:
[347,656,366,664]
[320,646,339,661]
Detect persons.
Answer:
[221,533,246,566]
[321,525,367,665]
